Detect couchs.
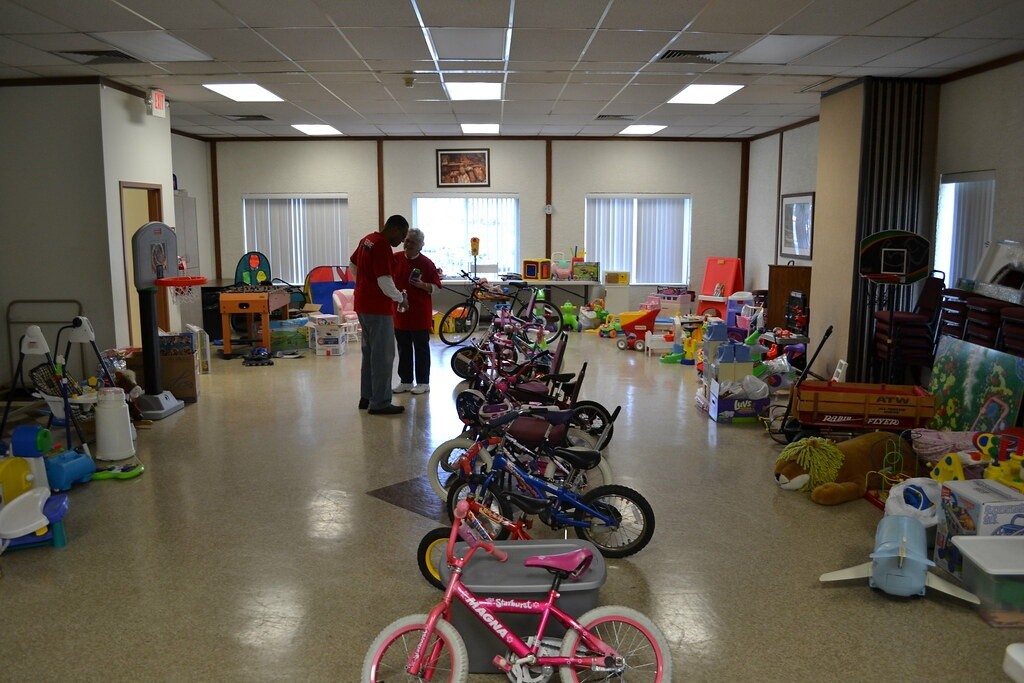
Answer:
[333,289,358,323]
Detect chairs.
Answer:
[872,270,1024,384]
[346,321,359,344]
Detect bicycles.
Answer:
[438,268,563,345]
[417,304,658,591]
[360,498,678,683]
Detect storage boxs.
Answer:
[933,478,1024,582]
[438,539,607,674]
[306,313,346,355]
[123,322,210,402]
[792,379,936,434]
[950,535,1024,628]
[702,340,768,423]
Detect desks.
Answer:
[433,280,600,330]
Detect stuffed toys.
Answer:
[772,430,915,507]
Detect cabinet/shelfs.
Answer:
[767,264,812,333]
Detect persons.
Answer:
[390,229,442,394]
[348,216,411,414]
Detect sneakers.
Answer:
[411,383,430,394]
[392,383,413,393]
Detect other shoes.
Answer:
[358,399,405,415]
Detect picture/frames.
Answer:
[780,191,815,260]
[436,147,490,187]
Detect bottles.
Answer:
[397,289,407,313]
[408,268,421,284]
[178,258,185,275]
